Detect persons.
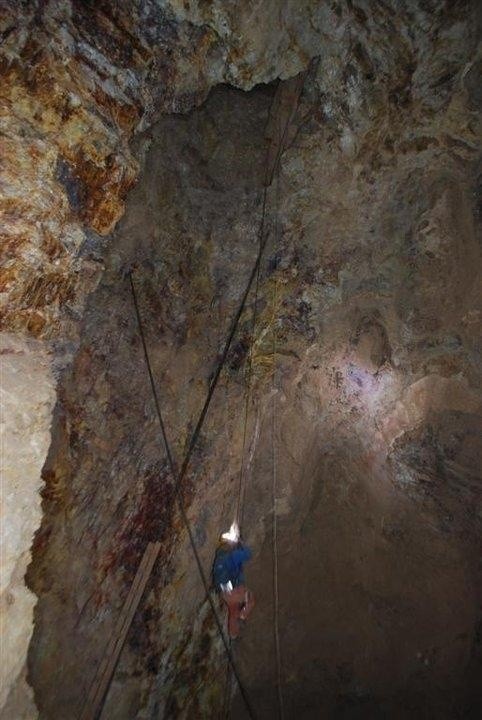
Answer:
[211,523,256,640]
